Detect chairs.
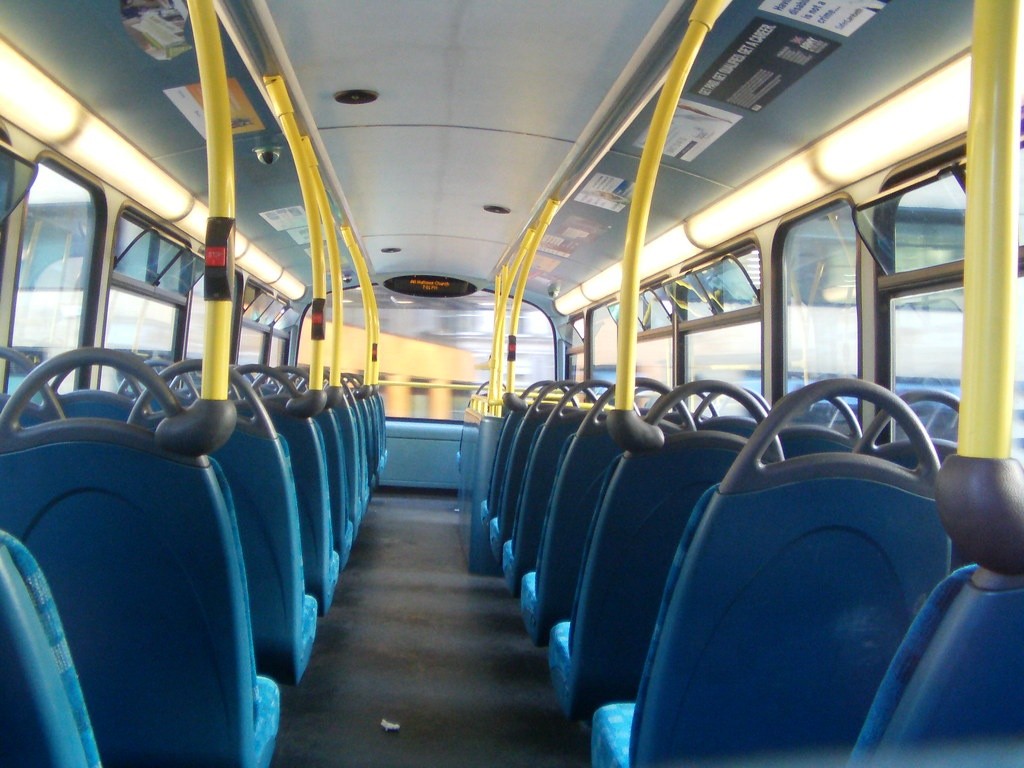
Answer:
[0,348,1024,768]
